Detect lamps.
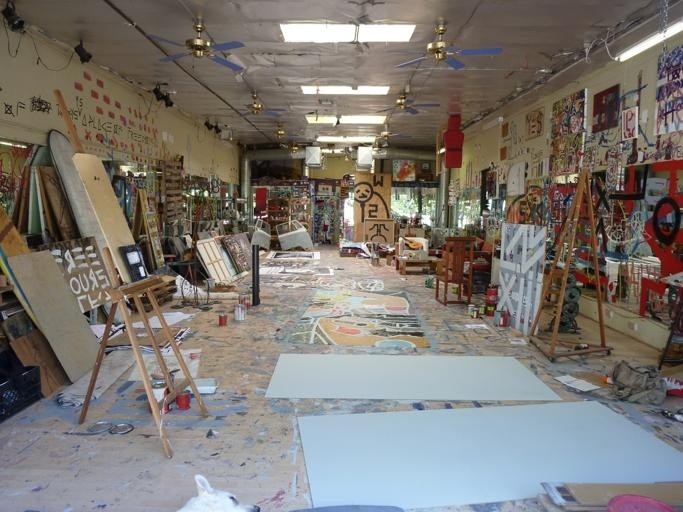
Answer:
[205,119,214,129]
[2,1,24,32]
[74,39,93,64]
[215,125,221,133]
[384,25,503,71]
[153,83,164,100]
[615,21,683,67]
[164,93,174,108]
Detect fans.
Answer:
[219,94,288,119]
[376,124,398,140]
[376,96,441,116]
[258,122,299,140]
[288,138,300,153]
[147,25,247,73]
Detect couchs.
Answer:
[399,237,428,256]
[442,236,496,272]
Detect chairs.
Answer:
[436,237,476,306]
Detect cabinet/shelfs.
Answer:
[315,196,341,244]
[250,179,316,248]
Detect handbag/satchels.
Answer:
[612,357,668,404]
